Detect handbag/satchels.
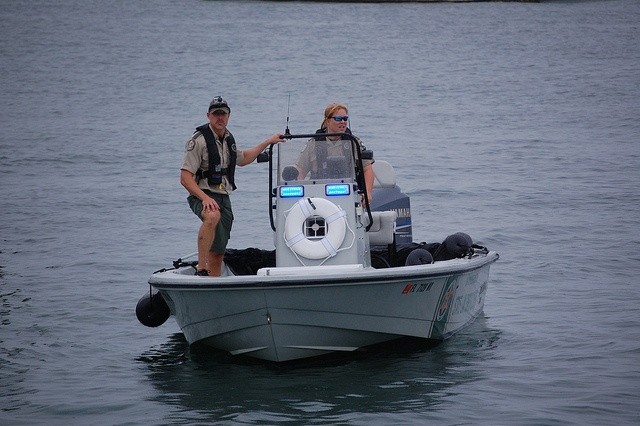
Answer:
[397,241,440,267]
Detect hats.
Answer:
[209,96,229,114]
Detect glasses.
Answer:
[328,115,348,121]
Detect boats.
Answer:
[136,91,500,362]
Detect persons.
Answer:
[294,103,375,209]
[180,95,285,275]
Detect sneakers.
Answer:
[196,270,208,276]
